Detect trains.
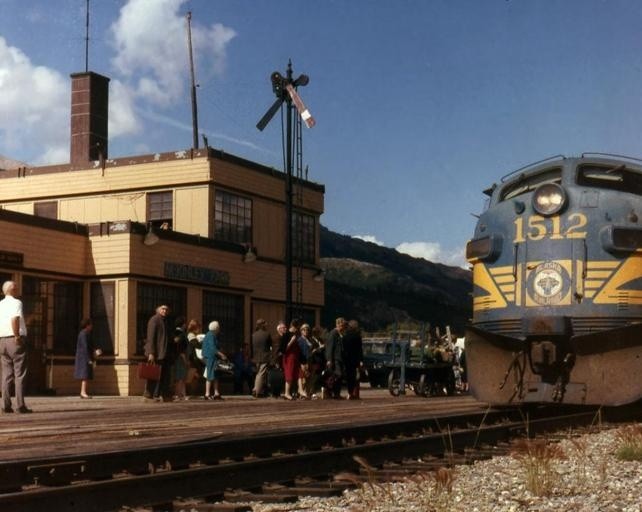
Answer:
[464,153,642,407]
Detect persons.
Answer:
[72,315,96,399]
[425,335,470,394]
[248,315,364,402]
[0,281,35,414]
[140,304,233,401]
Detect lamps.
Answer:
[143,222,163,245]
[312,268,330,282]
[243,247,259,264]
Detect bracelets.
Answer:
[15,336,23,341]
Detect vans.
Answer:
[312,339,411,388]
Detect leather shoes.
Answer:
[204,395,223,400]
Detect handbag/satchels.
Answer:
[138,361,161,382]
[215,360,234,375]
